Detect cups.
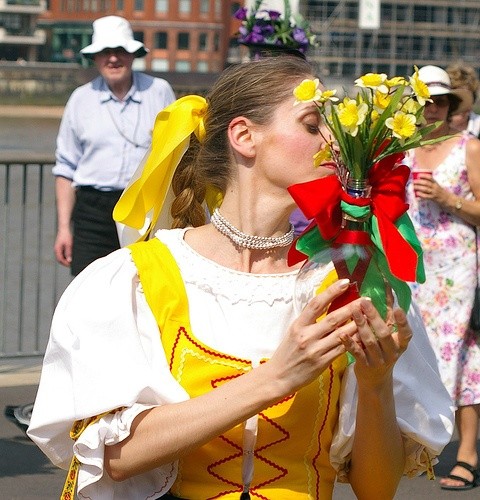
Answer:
[412,169,433,200]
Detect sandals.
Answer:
[440,461,480,489]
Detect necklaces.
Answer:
[203,205,297,254]
[422,128,451,151]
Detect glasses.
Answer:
[422,99,450,108]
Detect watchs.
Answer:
[454,195,465,216]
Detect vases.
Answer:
[244,44,304,59]
[291,182,409,339]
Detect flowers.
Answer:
[294,65,460,177]
[233,9,316,47]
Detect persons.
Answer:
[394,64,480,492]
[25,49,415,500]
[444,57,480,137]
[48,11,176,278]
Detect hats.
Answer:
[408,65,464,111]
[79,14,150,58]
